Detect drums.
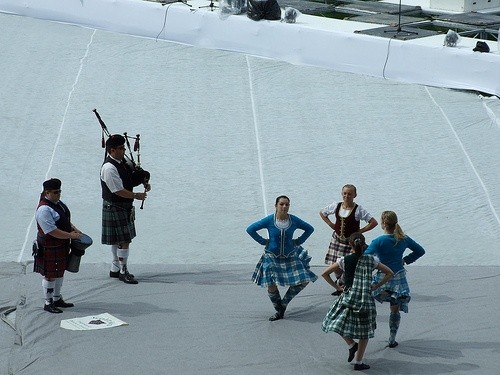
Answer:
[66,233,93,274]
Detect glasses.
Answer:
[116,146,124,150]
[49,190,61,194]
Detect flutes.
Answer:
[92,110,151,209]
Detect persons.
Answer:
[32,178,83,314]
[364,210,425,348]
[100,134,147,285]
[320,184,378,296]
[245,196,319,321]
[321,232,394,371]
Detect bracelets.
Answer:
[133,193,136,198]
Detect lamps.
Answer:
[443,29,461,47]
[220,0,299,23]
[473,40,491,52]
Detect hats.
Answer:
[42,178,61,191]
[106,135,125,147]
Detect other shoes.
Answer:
[331,291,343,296]
[119,271,138,284]
[109,270,134,278]
[269,312,283,321]
[334,276,342,283]
[44,303,63,313]
[347,342,359,362]
[354,363,370,371]
[389,341,398,348]
[53,298,74,308]
[278,300,285,319]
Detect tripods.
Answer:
[199,0,219,11]
[384,0,418,37]
[162,0,192,7]
